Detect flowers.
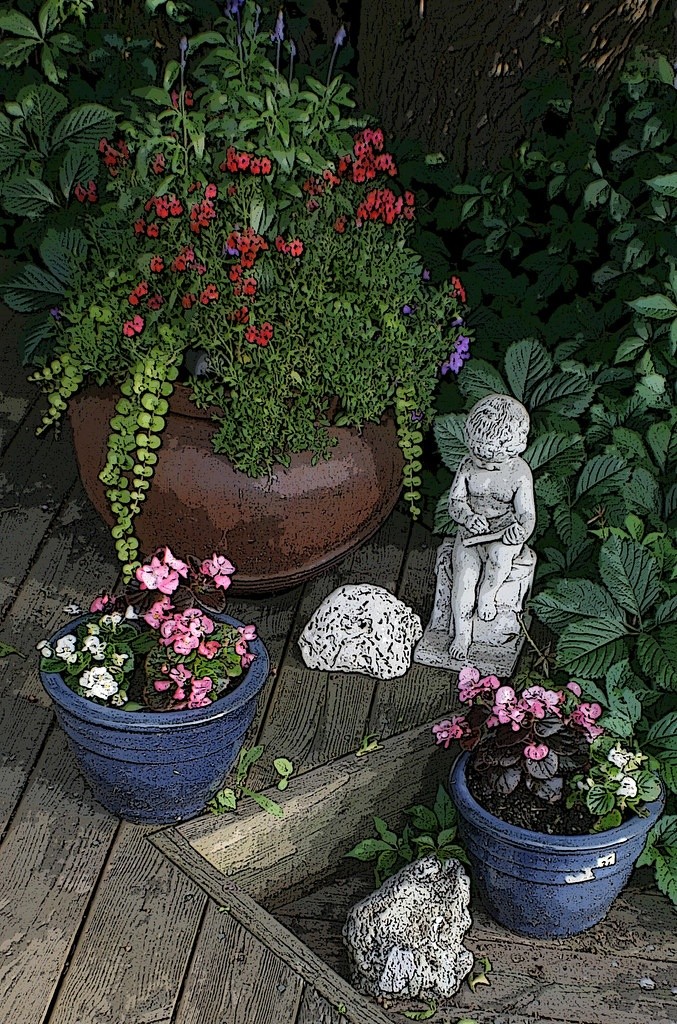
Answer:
[28,2,475,586]
[431,666,661,831]
[36,547,257,712]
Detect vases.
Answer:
[39,608,269,824]
[451,749,666,939]
[70,373,406,589]
